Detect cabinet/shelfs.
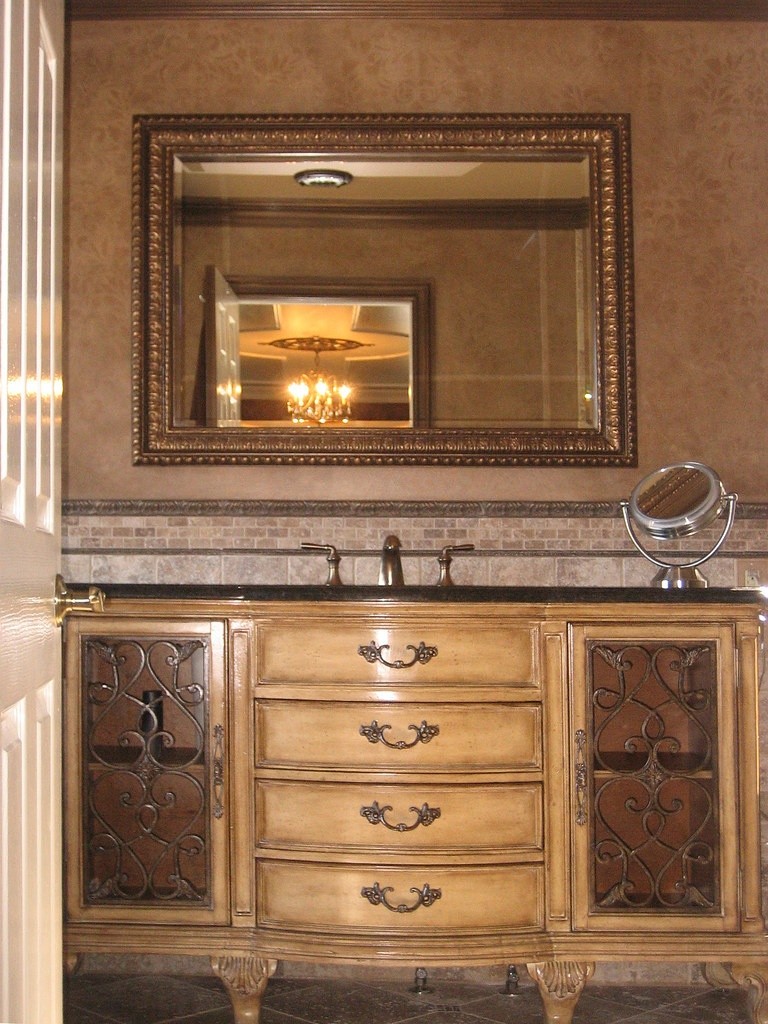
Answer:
[61,597,767,966]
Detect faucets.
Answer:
[378,535,405,585]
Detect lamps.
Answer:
[268,338,363,425]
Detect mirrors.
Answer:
[129,113,639,466]
